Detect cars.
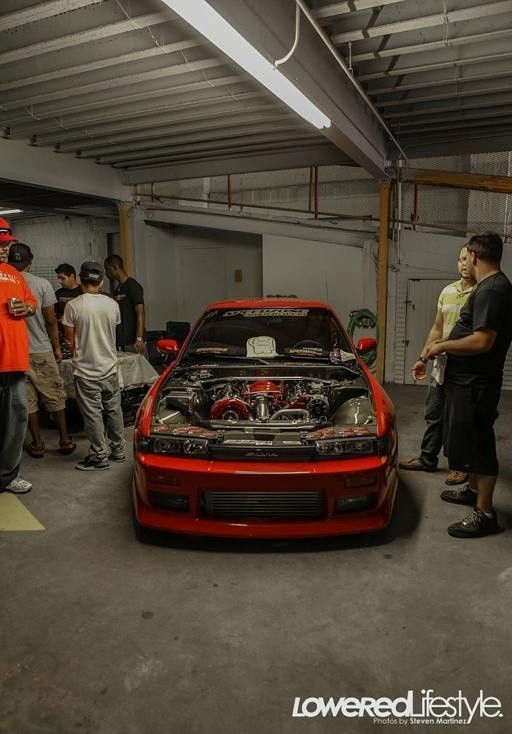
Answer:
[131,297,401,542]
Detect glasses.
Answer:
[456,258,468,265]
[0,228,12,236]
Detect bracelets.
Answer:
[418,357,428,364]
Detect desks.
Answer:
[57,350,160,429]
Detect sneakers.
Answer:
[445,469,467,485]
[59,437,75,451]
[76,456,110,472]
[5,477,31,493]
[440,485,476,506]
[115,456,126,462]
[447,508,498,538]
[28,441,45,458]
[399,457,434,471]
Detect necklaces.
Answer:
[117,276,127,292]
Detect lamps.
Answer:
[163,0,331,134]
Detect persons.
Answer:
[427,232,512,538]
[7,243,76,459]
[54,263,83,359]
[398,243,477,485]
[0,217,36,494]
[104,254,145,355]
[62,262,126,472]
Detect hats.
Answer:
[8,243,31,271]
[78,261,104,279]
[0,217,17,243]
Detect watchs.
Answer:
[136,336,144,342]
